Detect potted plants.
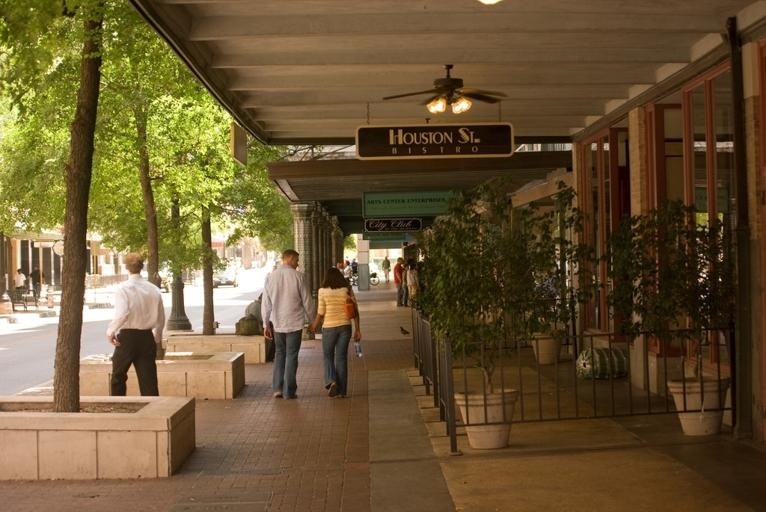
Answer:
[403,177,529,451]
[599,199,740,438]
[529,178,611,368]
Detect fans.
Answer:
[379,63,507,116]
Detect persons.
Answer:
[331,259,358,285]
[305,268,362,398]
[260,249,316,400]
[15,269,27,302]
[106,252,165,395]
[29,266,45,303]
[382,256,391,283]
[391,257,425,307]
[243,293,276,361]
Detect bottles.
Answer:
[353,338,362,358]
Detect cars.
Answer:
[140,263,170,293]
[213,267,239,288]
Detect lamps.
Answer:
[427,93,473,116]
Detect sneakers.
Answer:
[285,395,298,400]
[273,391,283,398]
[328,382,336,398]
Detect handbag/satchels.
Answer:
[345,302,357,319]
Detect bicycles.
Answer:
[351,273,379,286]
